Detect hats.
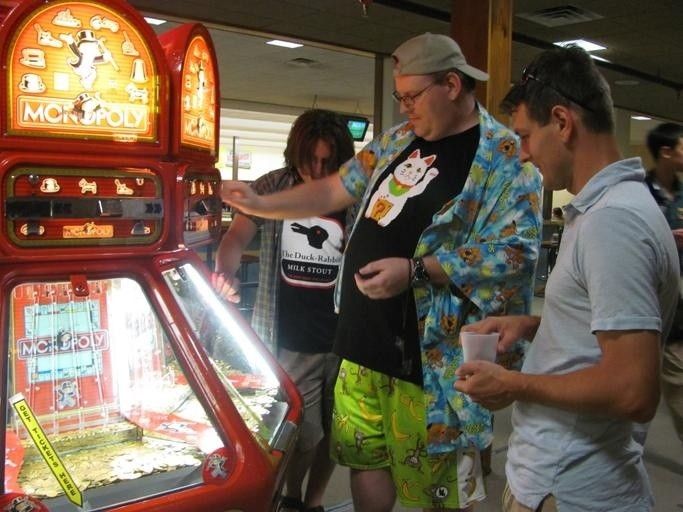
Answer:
[391,31,490,82]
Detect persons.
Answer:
[216,32,546,512]
[644,121,683,441]
[451,45,682,512]
[211,108,361,512]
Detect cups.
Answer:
[459,331,498,378]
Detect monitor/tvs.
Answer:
[344,115,370,142]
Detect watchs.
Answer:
[409,257,430,289]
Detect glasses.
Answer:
[520,61,595,112]
[391,72,447,104]
